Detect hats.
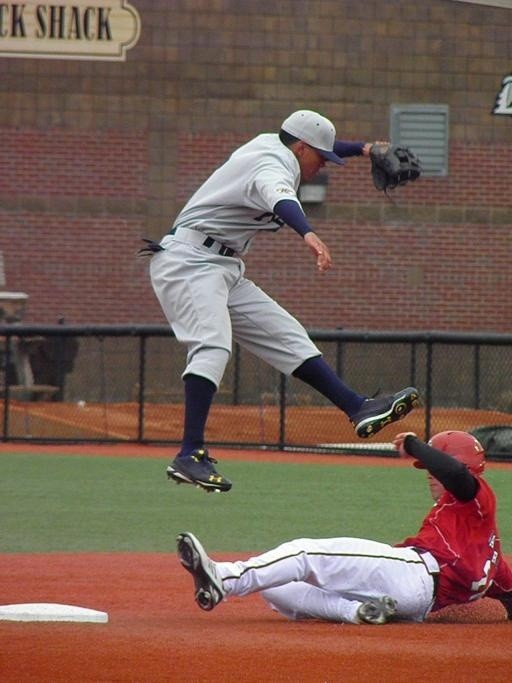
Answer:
[281,110,345,165]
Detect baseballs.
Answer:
[78,400,85,407]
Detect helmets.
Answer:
[414,431,486,476]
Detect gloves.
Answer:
[136,239,163,259]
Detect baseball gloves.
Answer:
[370,141,420,191]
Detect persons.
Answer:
[177,431,512,625]
[149,109,420,493]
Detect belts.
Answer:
[412,547,438,597]
[167,225,235,257]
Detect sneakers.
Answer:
[176,533,227,611]
[358,597,398,625]
[348,387,420,438]
[165,449,232,493]
